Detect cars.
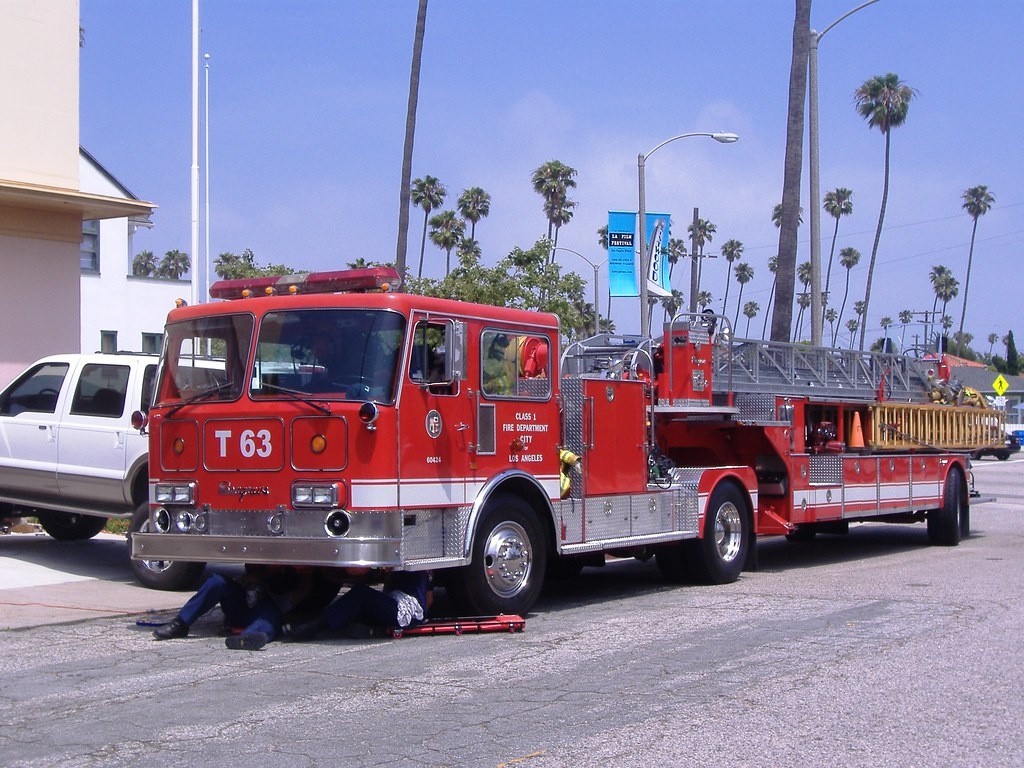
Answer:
[967,424,1021,461]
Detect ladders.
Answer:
[867,404,1007,450]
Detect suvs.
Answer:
[0,351,328,590]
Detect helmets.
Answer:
[305,319,339,348]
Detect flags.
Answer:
[646,214,672,296]
[607,210,641,297]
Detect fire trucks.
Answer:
[129,265,1012,618]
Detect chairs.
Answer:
[89,388,123,415]
[392,343,437,383]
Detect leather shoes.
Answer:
[153,614,190,640]
[225,633,268,650]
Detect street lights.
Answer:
[638,130,740,337]
[549,246,610,336]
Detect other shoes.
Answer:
[282,623,317,640]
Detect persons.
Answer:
[153,564,434,650]
